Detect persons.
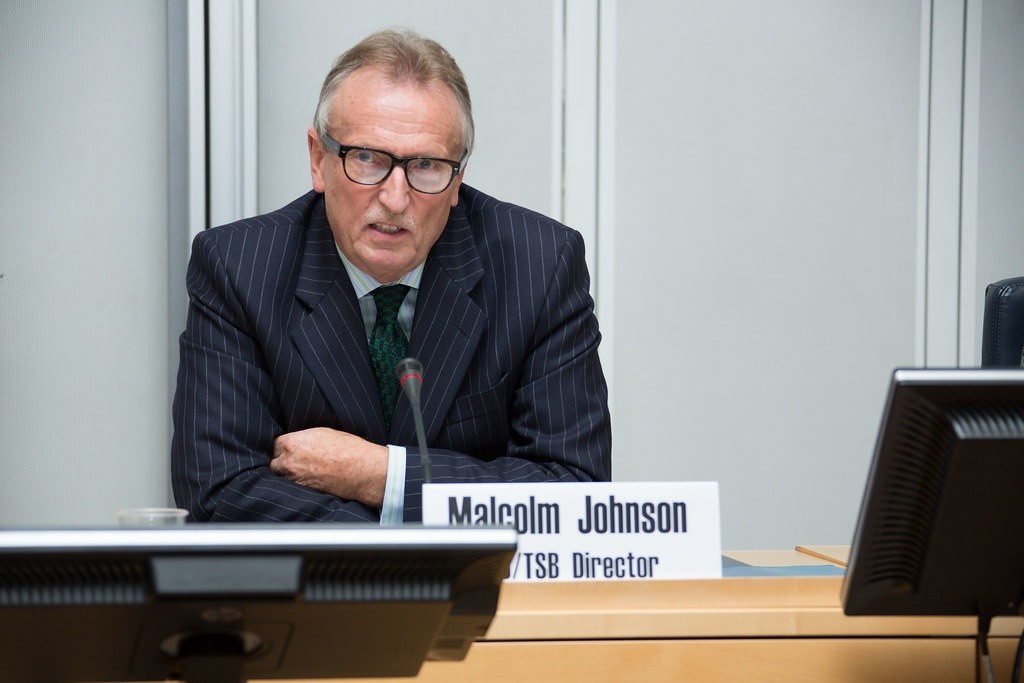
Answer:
[170,31,612,525]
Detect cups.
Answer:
[116,507,189,530]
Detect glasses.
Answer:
[323,132,468,194]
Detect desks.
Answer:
[256,548,1024,683]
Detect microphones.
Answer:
[394,358,431,483]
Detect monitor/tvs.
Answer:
[0,527,521,683]
[841,369,1024,619]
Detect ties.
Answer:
[368,284,412,431]
